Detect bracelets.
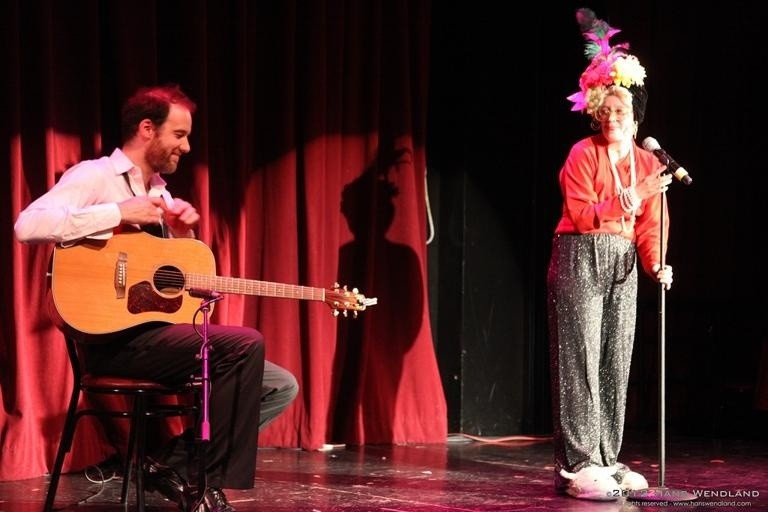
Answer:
[618,186,643,213]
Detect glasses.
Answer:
[594,106,632,121]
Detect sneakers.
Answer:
[608,461,649,492]
[557,462,623,501]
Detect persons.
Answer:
[536,9,676,495]
[11,88,300,510]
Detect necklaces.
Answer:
[608,140,641,237]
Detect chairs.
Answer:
[42,335,201,512]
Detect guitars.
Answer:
[45,223,366,339]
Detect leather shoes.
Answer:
[130,455,184,503]
[196,488,236,512]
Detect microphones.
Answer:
[642,136,693,185]
[189,287,220,299]
